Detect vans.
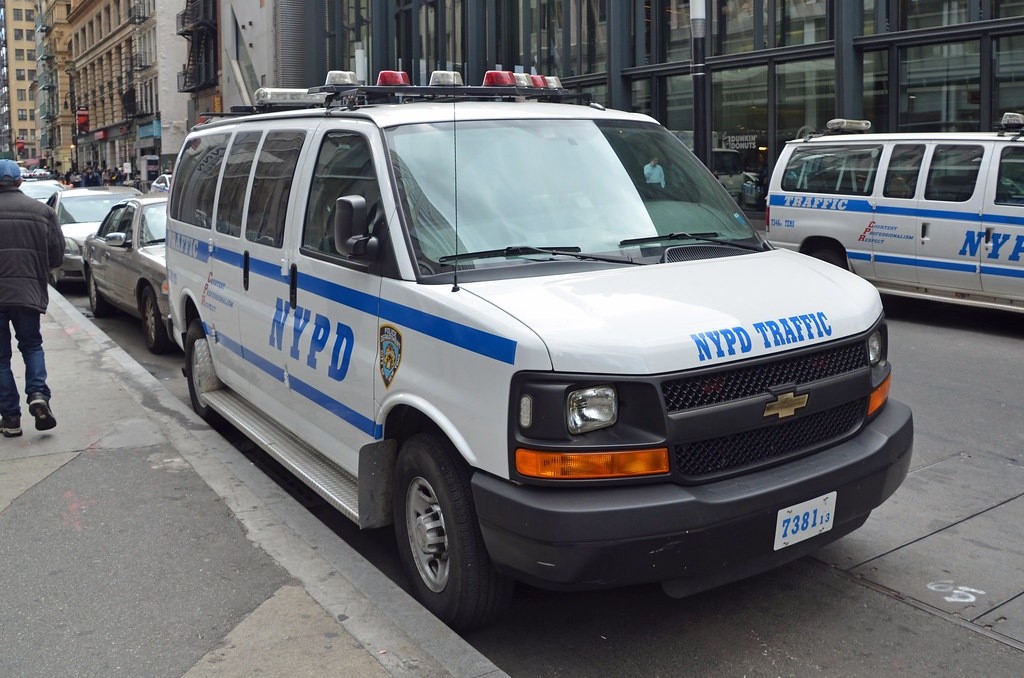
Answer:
[765,110,1023,320]
[166,71,911,618]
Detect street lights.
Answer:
[70,144,79,170]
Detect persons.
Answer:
[644,155,665,198]
[0,158,67,438]
[17,153,142,188]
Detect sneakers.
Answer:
[0,414,23,436]
[27,392,57,430]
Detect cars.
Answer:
[19,167,29,177]
[84,192,183,355]
[151,174,173,193]
[32,168,50,178]
[47,186,143,287]
[20,178,66,206]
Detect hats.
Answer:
[0,159,20,186]
